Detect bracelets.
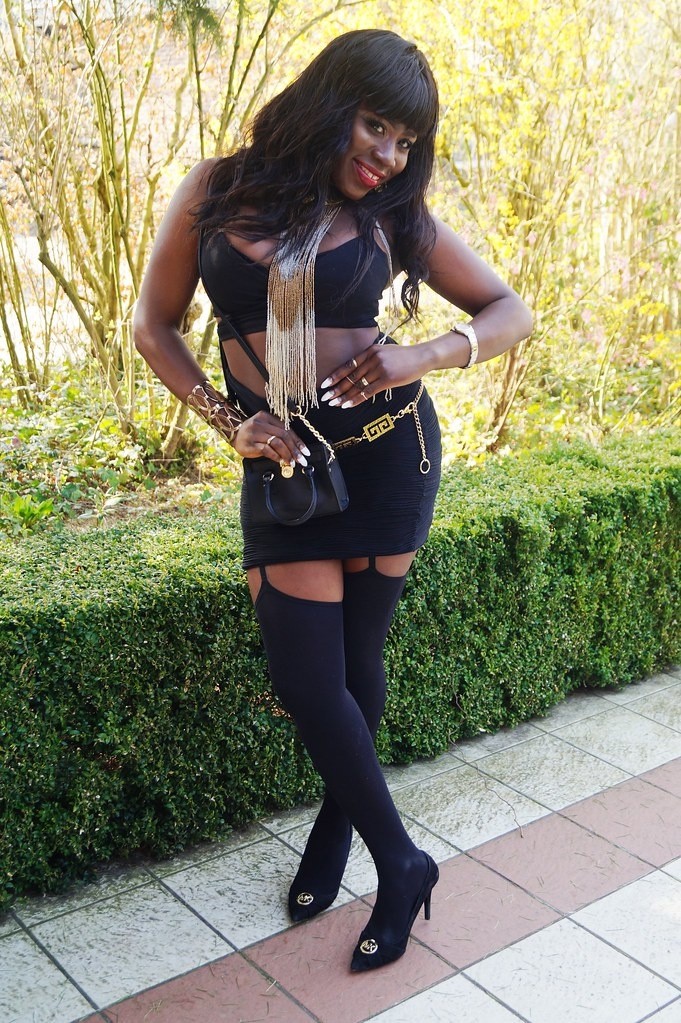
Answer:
[186,380,248,444]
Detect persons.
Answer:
[130,31,535,972]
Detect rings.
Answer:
[266,435,277,445]
[361,377,369,385]
[351,357,358,369]
[346,376,355,386]
[361,391,368,400]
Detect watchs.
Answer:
[449,322,478,369]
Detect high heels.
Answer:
[348,849,440,974]
[289,818,352,923]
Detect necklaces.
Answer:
[262,187,352,432]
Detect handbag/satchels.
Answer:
[243,437,351,526]
